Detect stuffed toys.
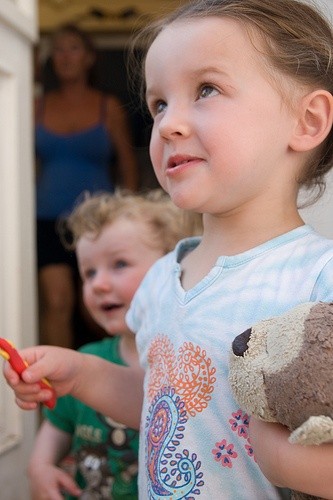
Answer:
[228,302,333,500]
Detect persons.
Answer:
[1,1,333,500]
[28,190,204,500]
[34,25,137,352]
